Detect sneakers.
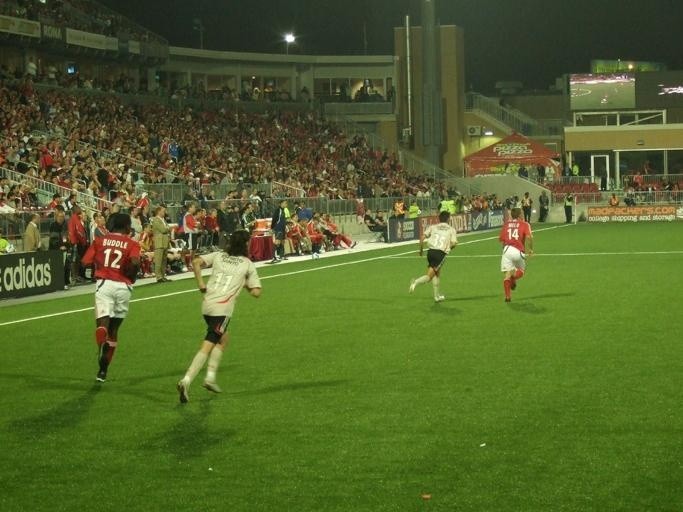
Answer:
[96,342,111,366]
[96,371,107,383]
[201,378,223,395]
[433,295,446,302]
[408,276,416,294]
[270,240,358,264]
[136,242,224,283]
[504,275,517,302]
[176,380,191,405]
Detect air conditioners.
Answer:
[465,125,482,137]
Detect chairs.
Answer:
[644,182,683,201]
[141,223,347,270]
[363,221,385,241]
[543,181,602,204]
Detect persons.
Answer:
[176,230,263,403]
[82,212,141,382]
